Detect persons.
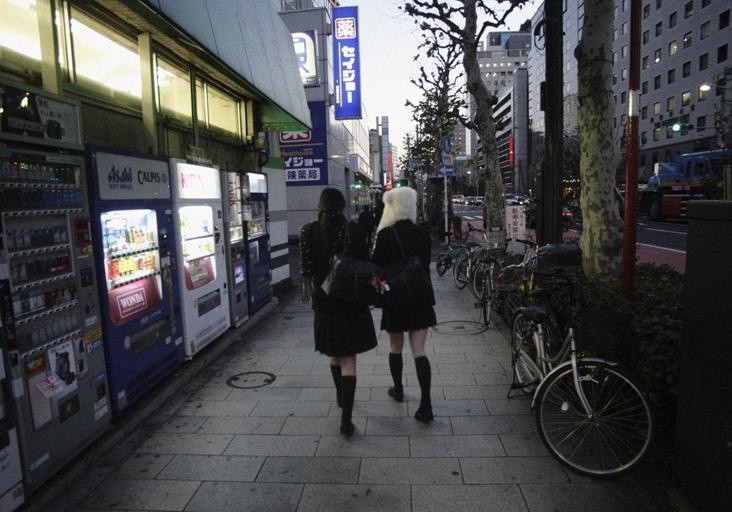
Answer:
[373,189,384,228]
[362,186,439,424]
[297,185,374,438]
[357,204,376,249]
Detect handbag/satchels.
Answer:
[320,223,429,313]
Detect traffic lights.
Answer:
[671,123,694,132]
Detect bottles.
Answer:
[4,223,80,320]
[102,220,156,280]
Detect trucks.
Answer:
[646,149,730,222]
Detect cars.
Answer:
[451,194,530,205]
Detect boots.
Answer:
[414,356,433,423]
[330,364,356,434]
[388,352,404,402]
